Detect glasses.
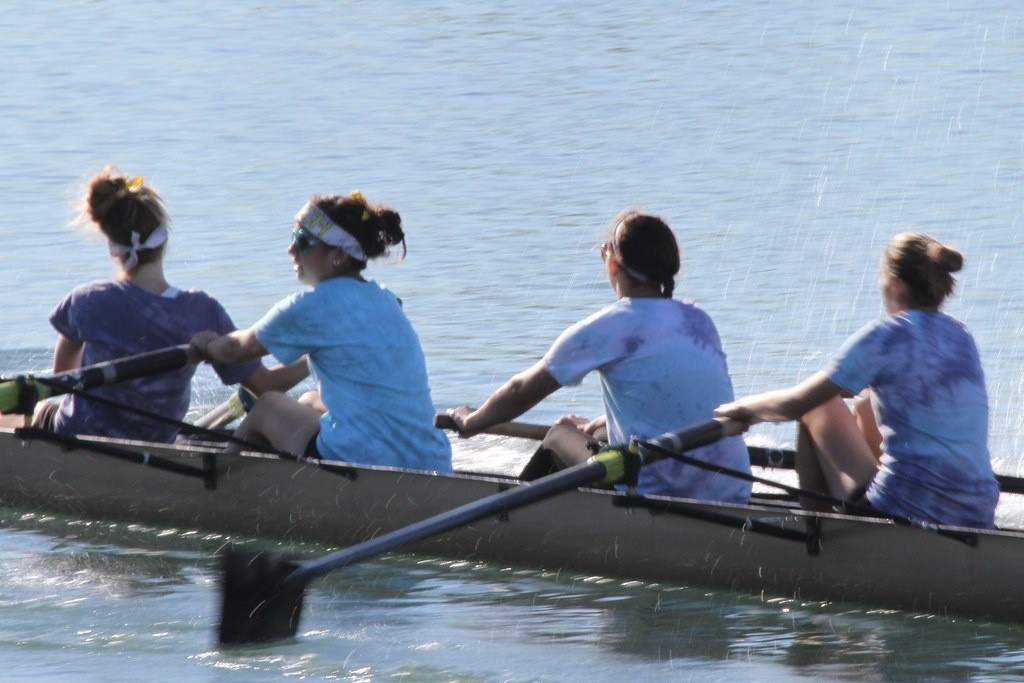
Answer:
[601,244,609,262]
[291,228,322,251]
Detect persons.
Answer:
[190,189,451,470]
[445,211,751,504]
[32,167,309,445]
[713,231,999,528]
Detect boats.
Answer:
[1,403,1024,632]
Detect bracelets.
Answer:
[205,336,218,357]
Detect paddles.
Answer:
[192,387,258,434]
[221,412,749,644]
[434,411,1023,501]
[0,343,204,420]
